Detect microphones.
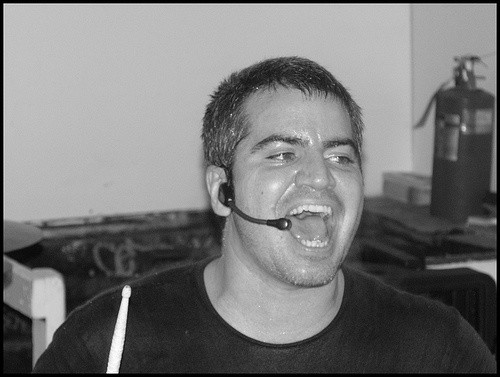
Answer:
[217,182,291,231]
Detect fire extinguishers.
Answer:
[426,55,495,223]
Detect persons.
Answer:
[33,57,497,373]
